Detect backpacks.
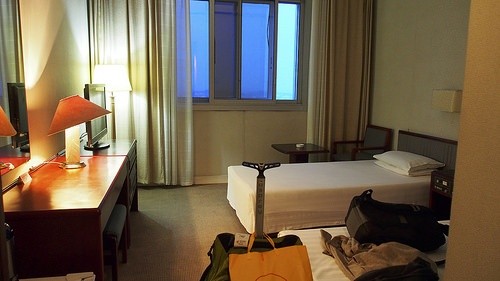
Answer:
[344,189,446,251]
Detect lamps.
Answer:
[0,106,17,137]
[93,64,132,139]
[441,88,462,113]
[47,95,111,169]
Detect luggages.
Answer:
[200,161,305,281]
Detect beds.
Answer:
[276,220,450,281]
[227,130,458,234]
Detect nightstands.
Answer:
[429,169,454,220]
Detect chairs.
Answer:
[333,124,393,163]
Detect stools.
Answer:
[104,205,128,281]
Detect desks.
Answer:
[2,139,140,281]
[1,145,30,190]
[272,143,330,163]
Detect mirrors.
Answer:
[0,3,32,195]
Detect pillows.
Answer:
[373,150,445,172]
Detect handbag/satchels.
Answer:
[229,232,313,281]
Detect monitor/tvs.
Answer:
[84,84,110,150]
[6,83,30,152]
